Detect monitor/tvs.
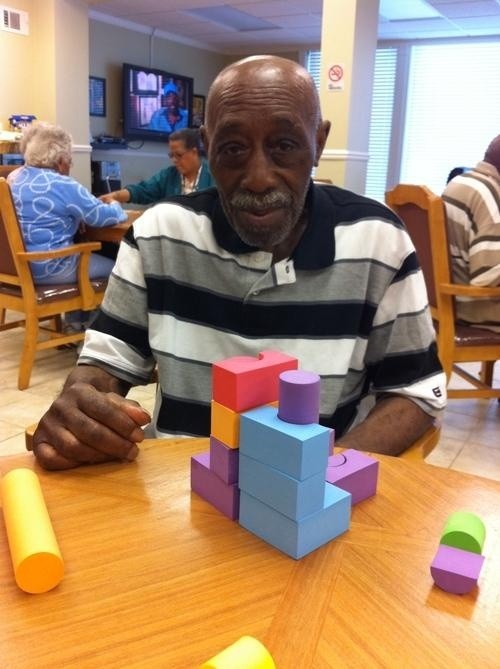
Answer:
[121,62,194,141]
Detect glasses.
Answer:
[167,148,193,157]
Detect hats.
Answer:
[164,83,179,98]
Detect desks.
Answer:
[75,208,150,248]
[0,432,500,669]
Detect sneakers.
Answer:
[57,324,84,349]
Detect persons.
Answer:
[33,54,448,472]
[6,123,128,352]
[98,127,218,206]
[442,132,499,338]
[147,85,186,134]
[6,125,47,187]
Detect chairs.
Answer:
[0,174,110,391]
[381,184,500,402]
